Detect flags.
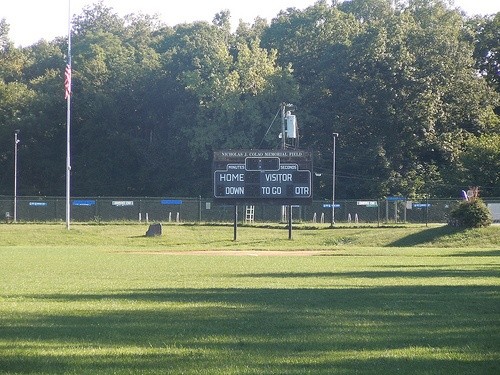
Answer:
[63,35,72,100]
